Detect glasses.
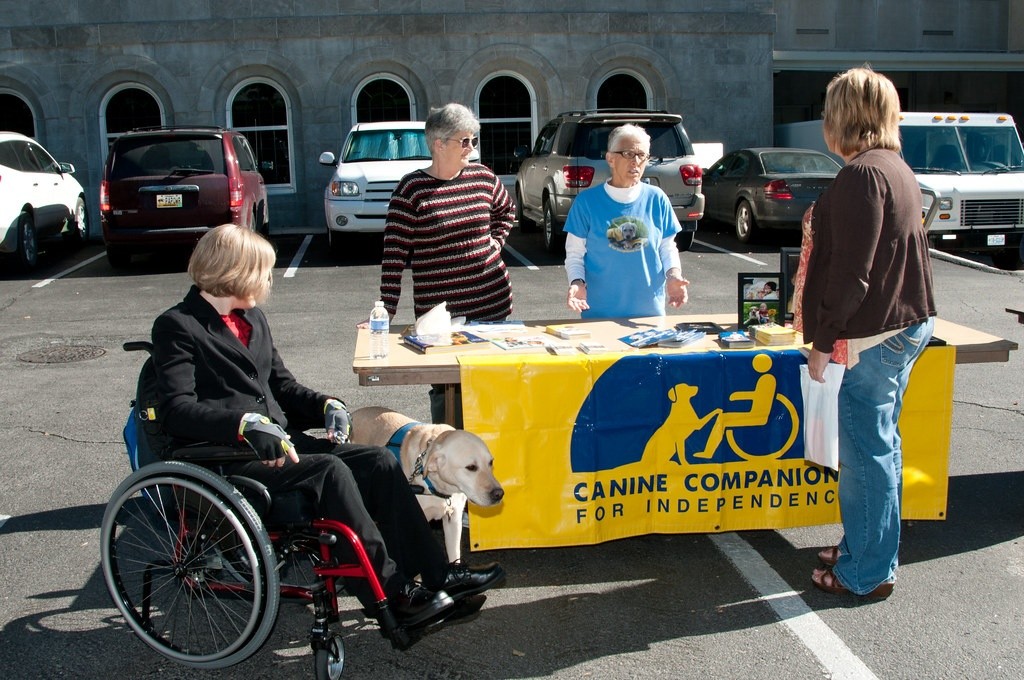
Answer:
[612,150,651,161]
[446,137,480,149]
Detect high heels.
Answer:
[812,567,896,598]
[818,546,844,565]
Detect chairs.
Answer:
[930,145,961,169]
[991,146,1019,168]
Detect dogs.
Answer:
[348,406,506,564]
[744,306,761,325]
[608,222,637,249]
[768,309,777,325]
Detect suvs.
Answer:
[100,124,269,267]
[0,129,89,268]
[318,121,479,249]
[515,107,705,253]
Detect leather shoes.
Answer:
[421,558,507,600]
[388,572,455,630]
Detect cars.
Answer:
[703,148,844,244]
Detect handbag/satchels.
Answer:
[797,362,846,471]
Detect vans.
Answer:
[776,110,1024,251]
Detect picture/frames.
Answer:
[781,248,802,320]
[738,272,786,330]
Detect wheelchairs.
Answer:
[100,339,488,680]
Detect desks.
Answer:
[352,310,1018,552]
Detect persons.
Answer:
[356,103,517,430]
[563,123,690,320]
[151,223,506,649]
[758,281,778,299]
[759,303,770,322]
[789,67,937,602]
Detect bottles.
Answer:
[370,301,389,360]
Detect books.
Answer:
[617,322,727,348]
[404,325,609,355]
[747,321,797,346]
[717,330,754,348]
